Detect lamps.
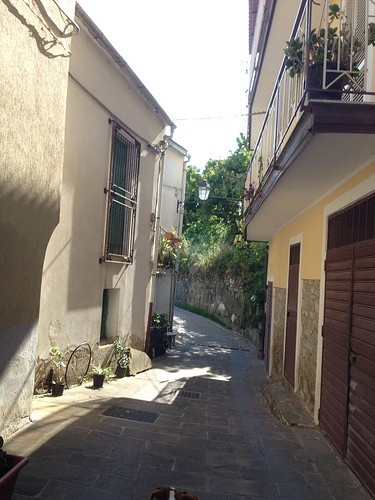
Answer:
[177,179,211,214]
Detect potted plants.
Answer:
[148,312,169,356]
[114,340,129,377]
[79,365,112,388]
[283,4,375,104]
[50,346,67,396]
